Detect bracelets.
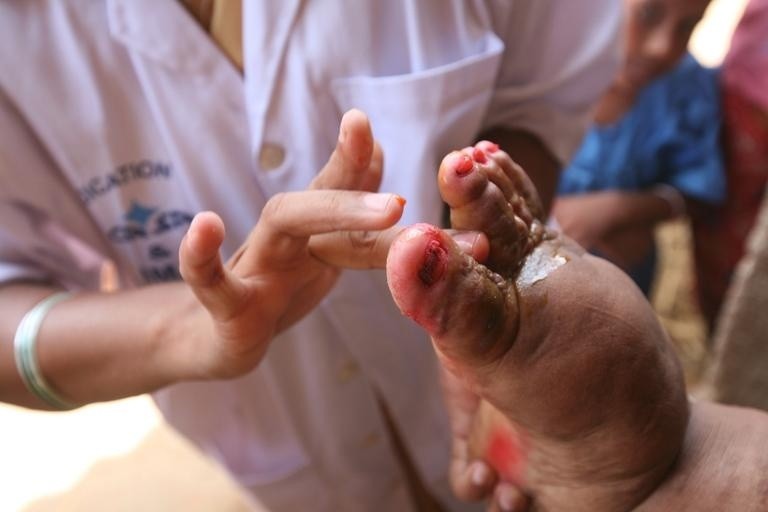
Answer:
[12,288,86,414]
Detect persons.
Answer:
[691,0,767,336]
[547,1,724,313]
[0,0,625,511]
[385,137,768,512]
